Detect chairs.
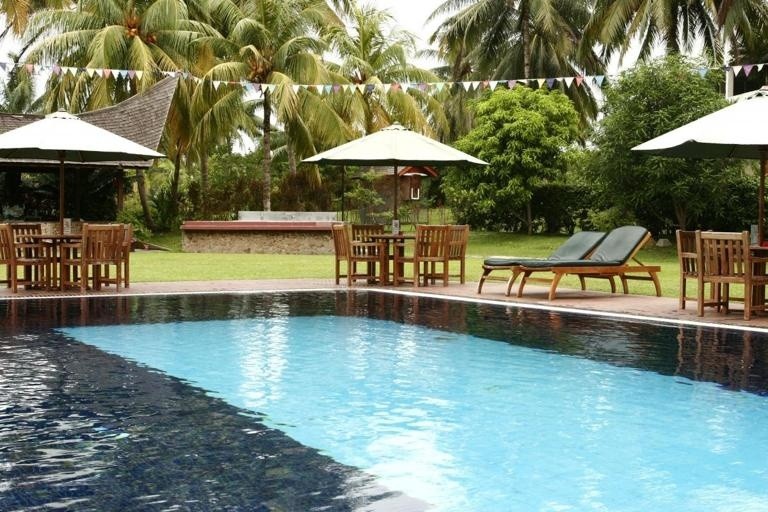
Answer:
[330,222,386,287]
[693,228,767,321]
[0,221,136,292]
[430,223,469,286]
[519,221,663,302]
[395,223,450,286]
[674,227,731,315]
[352,223,390,283]
[476,228,617,298]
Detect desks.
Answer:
[367,234,428,286]
[713,242,767,316]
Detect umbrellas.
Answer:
[301,120,491,219]
[630,92,768,245]
[0,107,168,234]
